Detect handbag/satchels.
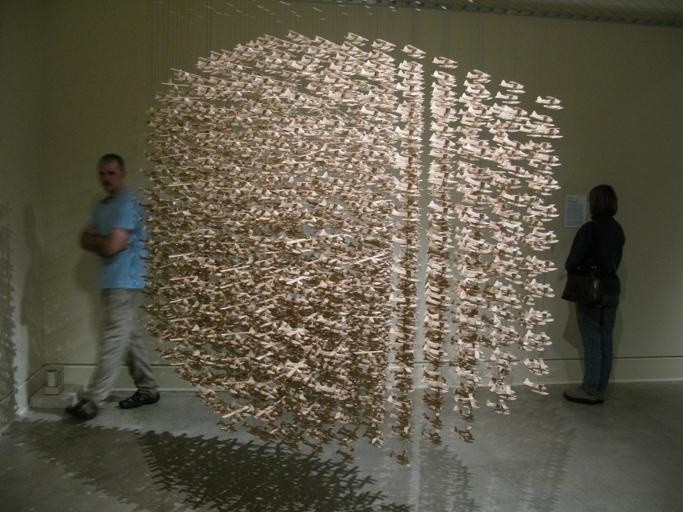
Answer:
[562,267,601,305]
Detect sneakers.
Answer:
[65,399,97,418]
[564,387,604,404]
[120,390,159,409]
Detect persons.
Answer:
[560,182,625,406]
[62,152,161,422]
